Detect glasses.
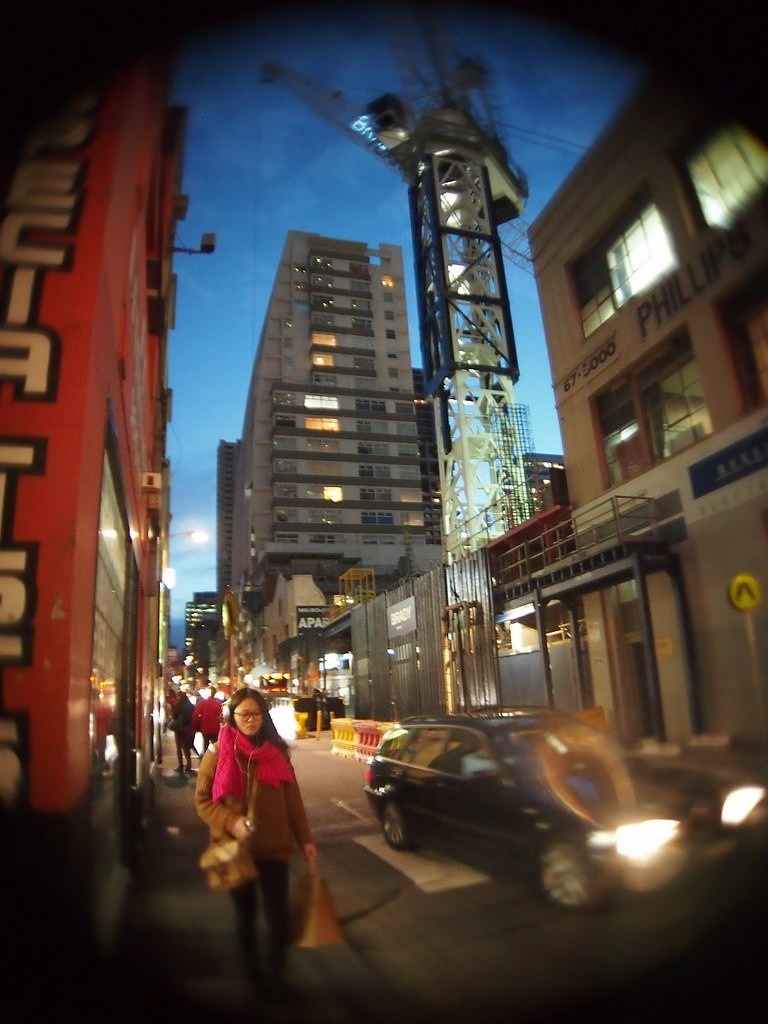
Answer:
[233,709,265,721]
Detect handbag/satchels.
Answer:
[168,717,176,731]
[290,857,344,947]
[199,839,259,893]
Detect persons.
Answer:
[162,696,173,736]
[166,689,176,705]
[195,687,315,994]
[172,692,196,774]
[193,686,223,750]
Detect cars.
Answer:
[364,706,768,917]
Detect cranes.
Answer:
[260,37,535,564]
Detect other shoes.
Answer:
[185,768,191,772]
[173,767,183,772]
